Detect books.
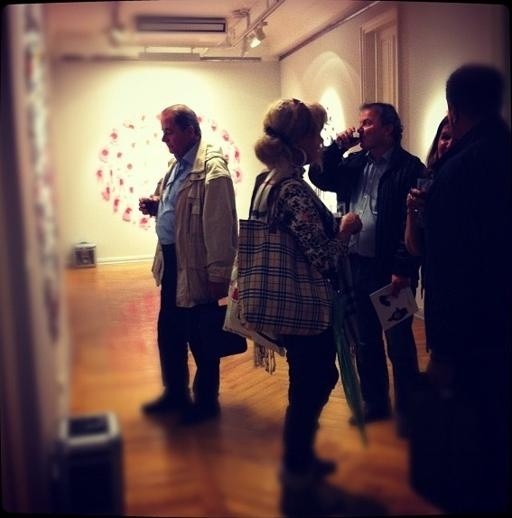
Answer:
[369,282,417,333]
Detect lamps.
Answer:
[137,45,201,64]
[249,27,266,48]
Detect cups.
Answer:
[331,127,361,150]
[141,193,156,219]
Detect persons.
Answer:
[306,103,431,437]
[421,62,512,517]
[137,104,238,424]
[248,98,385,517]
[405,114,459,352]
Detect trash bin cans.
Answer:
[56,410,123,518]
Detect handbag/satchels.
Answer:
[237,219,329,334]
[187,300,247,360]
